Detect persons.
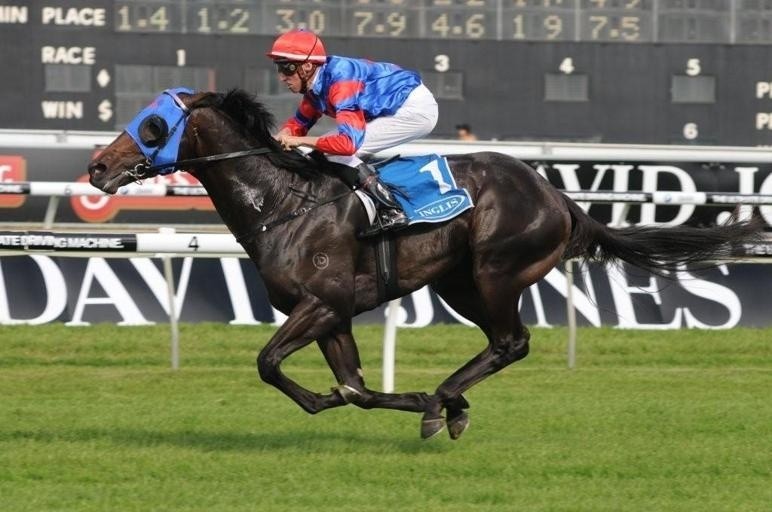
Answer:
[455,123,479,141]
[265,29,440,241]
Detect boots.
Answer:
[346,162,409,240]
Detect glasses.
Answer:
[277,61,306,76]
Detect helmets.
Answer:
[267,30,327,65]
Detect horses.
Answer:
[85,85,771,441]
